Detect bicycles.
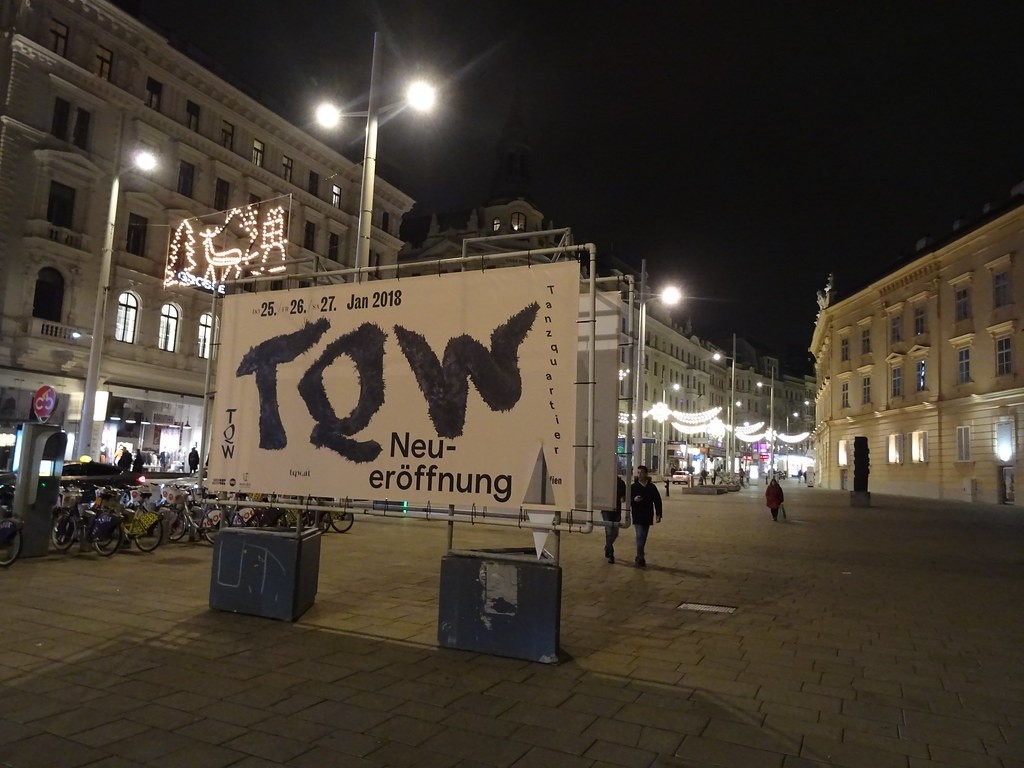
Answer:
[718,474,749,489]
[51,480,354,557]
[1,482,22,566]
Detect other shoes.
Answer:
[608,556,614,563]
[635,555,645,566]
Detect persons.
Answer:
[601,476,626,563]
[115,445,132,470]
[189,448,199,474]
[701,470,707,484]
[631,465,662,567]
[766,478,784,521]
[132,449,144,473]
[711,470,717,484]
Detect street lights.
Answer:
[633,286,682,477]
[714,353,735,477]
[787,412,799,478]
[726,402,741,472]
[659,383,679,476]
[757,382,774,480]
[75,149,158,465]
[314,79,435,279]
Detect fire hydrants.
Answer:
[664,480,669,496]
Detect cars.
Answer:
[672,471,689,484]
[777,471,785,480]
[62,461,145,488]
